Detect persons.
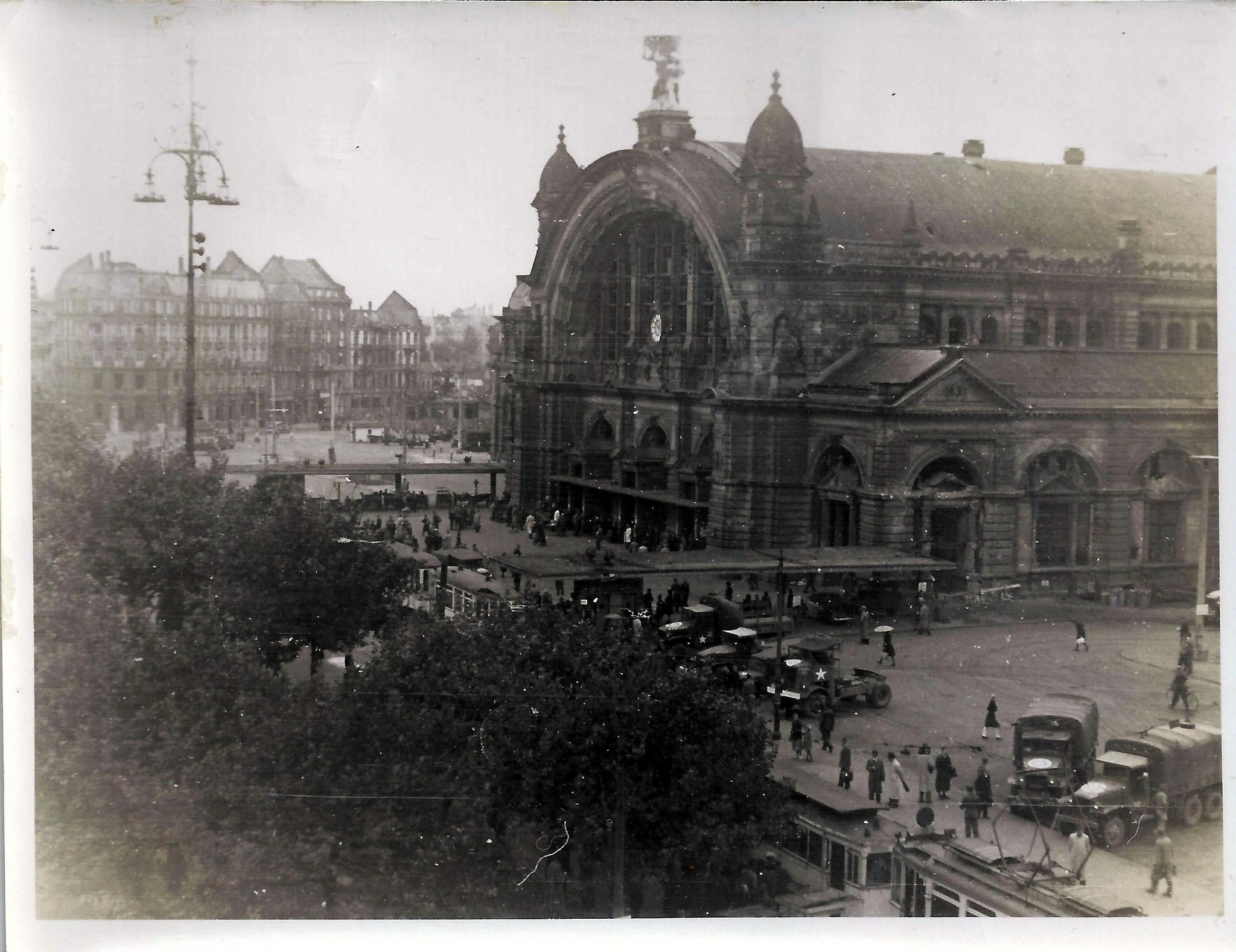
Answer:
[914,590,931,636]
[860,607,869,644]
[1145,827,1177,897]
[1176,616,1195,675]
[982,692,1003,740]
[973,757,993,819]
[789,704,835,763]
[555,567,818,699]
[866,750,885,804]
[876,631,895,667]
[959,785,982,838]
[837,737,851,790]
[154,420,707,608]
[917,743,953,803]
[841,571,883,603]
[1170,665,1191,712]
[886,751,904,808]
[1067,822,1090,885]
[1074,621,1089,651]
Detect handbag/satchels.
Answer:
[840,768,852,782]
[879,649,890,661]
[1076,636,1086,645]
[950,766,958,778]
[926,754,934,774]
[474,523,481,529]
[987,705,998,712]
[424,518,431,526]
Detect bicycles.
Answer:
[1164,683,1200,712]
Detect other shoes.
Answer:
[982,735,989,739]
[829,745,834,753]
[996,737,1004,740]
[891,663,896,667]
[876,661,883,665]
[938,793,950,800]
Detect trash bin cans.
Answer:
[1101,592,1109,604]
[1118,589,1127,607]
[1136,589,1143,607]
[1109,589,1118,607]
[1127,589,1136,608]
[1140,590,1149,609]
[1146,590,1152,608]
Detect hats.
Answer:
[1153,825,1166,837]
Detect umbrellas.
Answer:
[874,626,893,632]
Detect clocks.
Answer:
[649,313,662,341]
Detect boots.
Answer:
[1163,881,1173,898]
[1146,880,1159,894]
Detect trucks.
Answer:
[1010,693,1095,823]
[1060,718,1220,852]
[648,596,895,717]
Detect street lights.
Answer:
[1190,454,1220,633]
[140,106,242,465]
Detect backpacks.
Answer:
[434,516,442,522]
